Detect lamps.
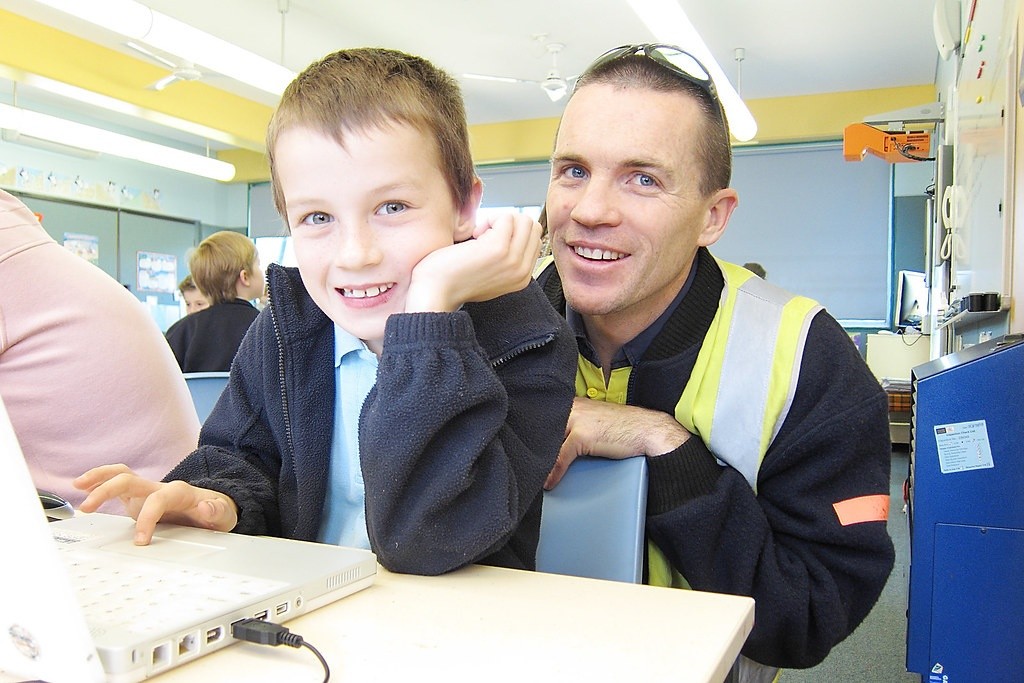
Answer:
[625,0,758,144]
[0,79,236,183]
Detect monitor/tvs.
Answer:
[893,270,929,328]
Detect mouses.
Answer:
[36,488,75,522]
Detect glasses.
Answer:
[574,43,731,160]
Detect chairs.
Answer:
[531,450,649,585]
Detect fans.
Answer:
[124,41,204,93]
[464,43,586,102]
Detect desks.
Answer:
[0,506,756,683]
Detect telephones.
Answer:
[941,184,954,230]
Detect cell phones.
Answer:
[878,330,894,335]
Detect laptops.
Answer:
[0,403,377,683]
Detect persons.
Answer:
[0,187,201,517]
[74,47,577,577]
[165,231,269,373]
[477,55,896,683]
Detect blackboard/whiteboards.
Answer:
[934,0,1018,328]
[247,137,896,332]
